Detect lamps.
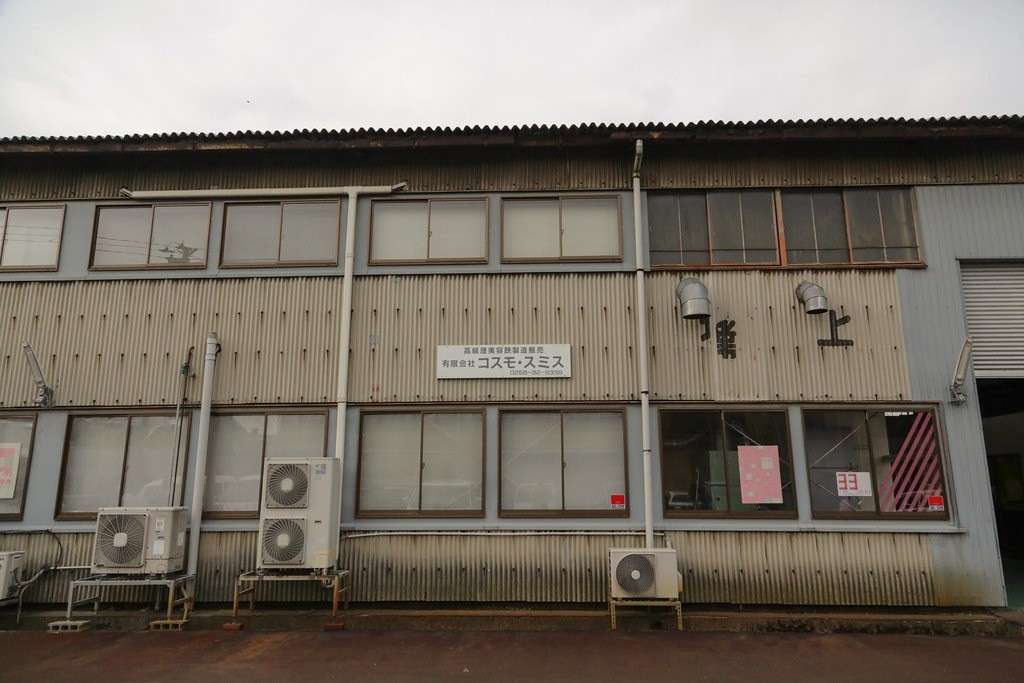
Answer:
[948,337,974,405]
[23,341,53,406]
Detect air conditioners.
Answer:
[256,457,341,569]
[91,506,189,575]
[0,551,26,599]
[607,548,679,599]
